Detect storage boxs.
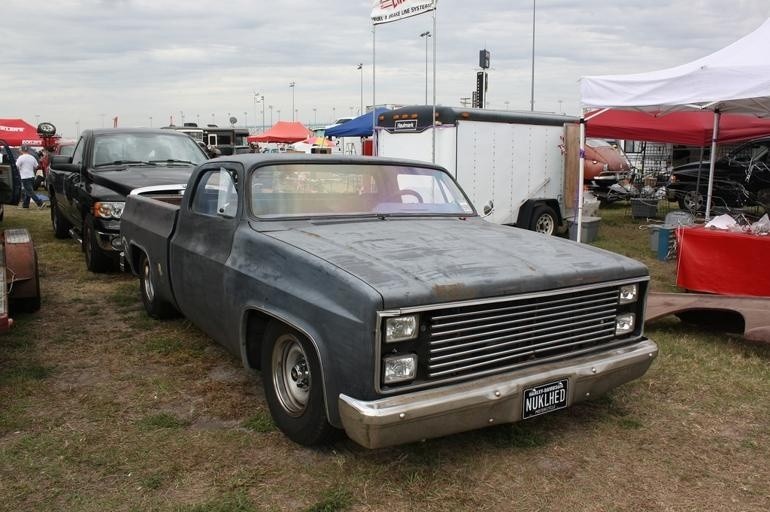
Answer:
[566,216,602,243]
[631,198,658,219]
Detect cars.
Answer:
[0,139,22,221]
[665,137,770,214]
[52,138,79,158]
[583,136,632,185]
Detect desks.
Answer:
[675,225,770,296]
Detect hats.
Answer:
[21,144,29,150]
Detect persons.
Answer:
[15,144,46,211]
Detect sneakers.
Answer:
[39,202,45,209]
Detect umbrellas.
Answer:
[303,137,336,149]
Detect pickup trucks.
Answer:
[118,151,661,449]
[46,127,236,274]
